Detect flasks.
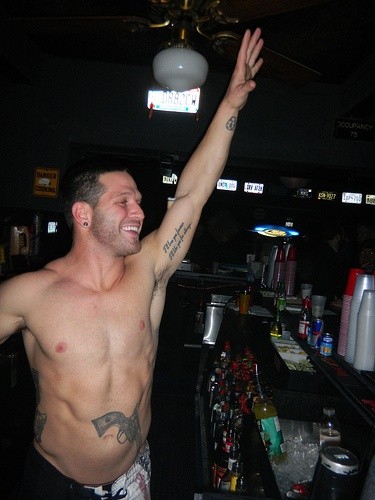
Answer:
[310,444,360,500]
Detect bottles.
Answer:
[268,309,282,339]
[297,295,311,340]
[273,281,286,314]
[319,407,342,448]
[208,353,286,496]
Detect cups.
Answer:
[301,283,312,299]
[265,245,296,296]
[337,269,375,372]
[239,289,250,315]
[311,294,326,319]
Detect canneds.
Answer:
[319,333,334,356]
[307,317,324,348]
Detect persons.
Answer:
[0,27,264,500]
[319,222,375,307]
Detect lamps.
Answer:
[124,0,242,93]
